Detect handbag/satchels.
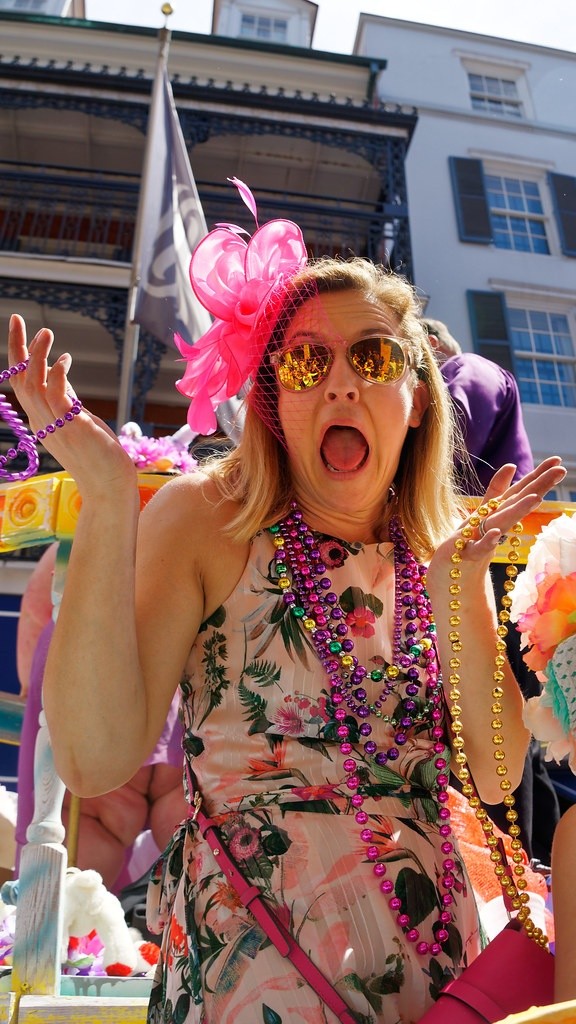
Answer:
[413,914,555,1023]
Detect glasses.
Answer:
[270,335,417,395]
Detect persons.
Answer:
[0,259,567,1023]
[420,318,561,874]
[353,350,397,382]
[18,540,196,892]
[280,358,327,390]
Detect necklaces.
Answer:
[0,359,83,481]
[272,505,456,955]
[454,499,547,946]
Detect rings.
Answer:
[479,520,507,545]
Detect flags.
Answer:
[130,58,218,356]
